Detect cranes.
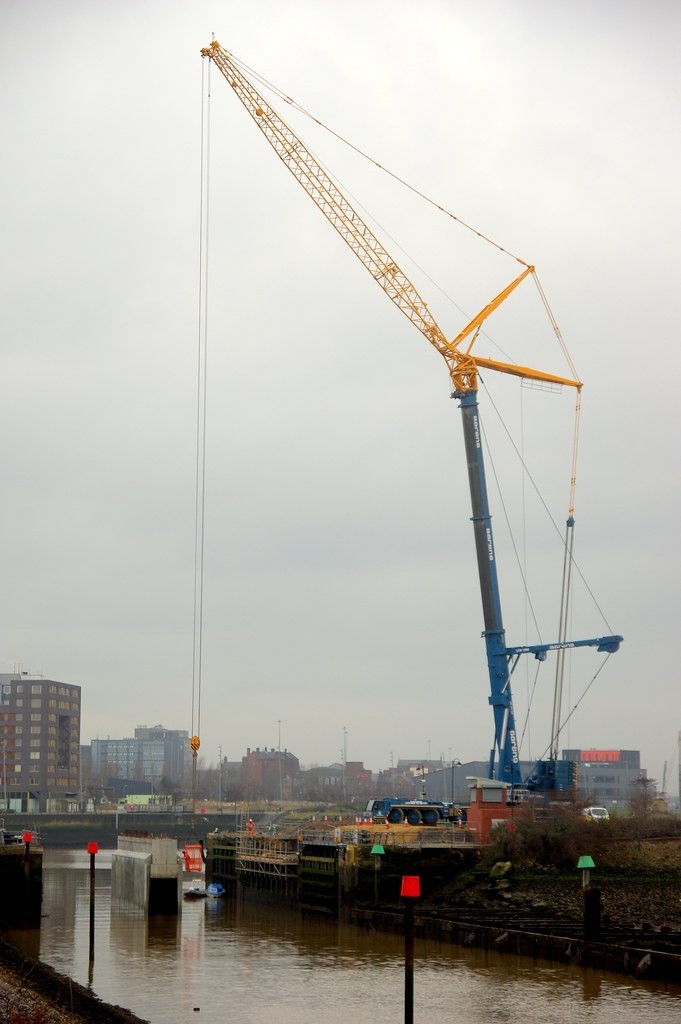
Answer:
[183,32,623,826]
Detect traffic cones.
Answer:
[355,814,374,826]
[383,816,391,828]
[312,816,316,823]
[338,815,342,822]
[324,815,328,824]
[404,817,409,828]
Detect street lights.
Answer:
[416,764,426,799]
[452,757,463,821]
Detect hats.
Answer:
[249,819,253,822]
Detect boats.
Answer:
[205,883,224,898]
[183,879,206,898]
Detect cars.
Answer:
[587,807,610,822]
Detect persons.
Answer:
[247,819,255,836]
[181,850,191,872]
